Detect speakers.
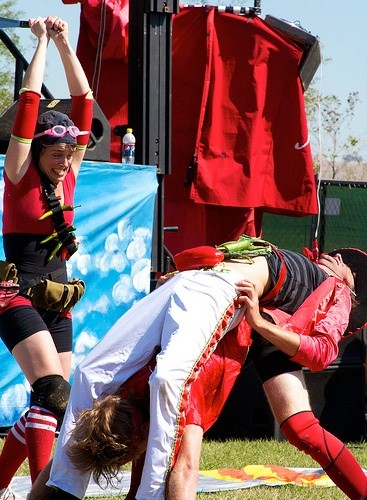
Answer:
[0,97,111,162]
[269,326,367,447]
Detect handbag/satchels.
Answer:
[25,277,85,314]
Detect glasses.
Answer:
[36,124,80,139]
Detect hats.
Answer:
[325,248,367,339]
[34,110,77,154]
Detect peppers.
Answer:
[174,246,225,271]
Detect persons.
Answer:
[0,16,96,500]
[26,234,367,500]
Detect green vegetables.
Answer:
[215,234,271,262]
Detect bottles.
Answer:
[122,128,136,165]
